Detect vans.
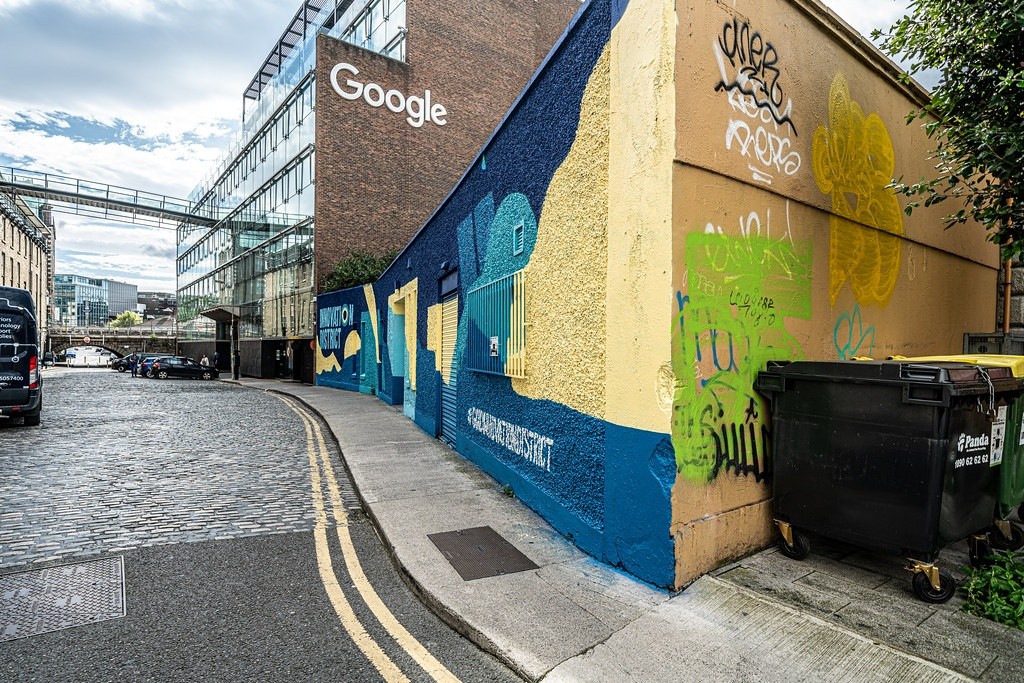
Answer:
[0,285,43,426]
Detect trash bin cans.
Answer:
[850,355,1024,522]
[753,360,1024,565]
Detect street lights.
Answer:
[214,279,237,380]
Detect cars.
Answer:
[85,345,93,350]
[64,350,77,358]
[43,352,58,362]
[149,356,219,380]
[111,352,147,373]
[96,347,104,352]
[139,357,180,378]
[100,348,112,356]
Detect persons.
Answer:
[200,354,209,366]
[213,351,220,369]
[52,351,56,366]
[130,351,139,377]
